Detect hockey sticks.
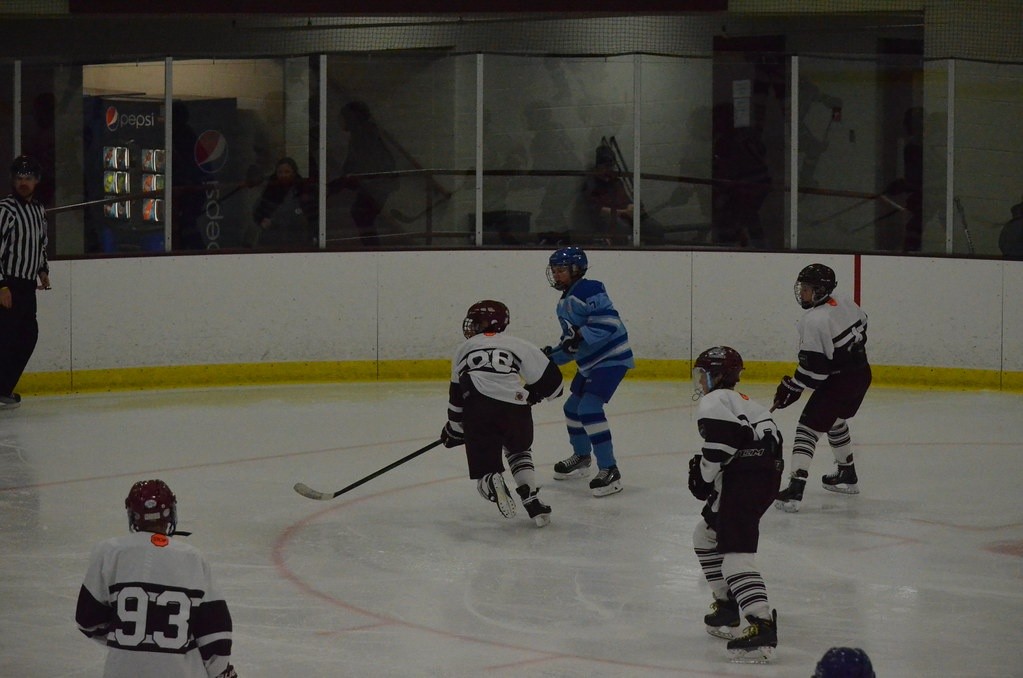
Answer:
[768,396,784,415]
[390,187,460,225]
[293,438,445,501]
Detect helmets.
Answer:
[694,346,743,388]
[546,247,588,291]
[11,156,41,178]
[125,479,178,536]
[793,263,838,308]
[463,300,510,339]
[811,647,875,678]
[596,146,617,164]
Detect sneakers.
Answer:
[477,472,516,518]
[821,452,860,494]
[704,589,741,639]
[589,465,623,496]
[775,468,809,513]
[728,608,777,664]
[516,485,552,526]
[0,393,21,410]
[553,453,591,480]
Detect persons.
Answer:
[888,106,923,256]
[328,100,397,249]
[812,647,877,678]
[538,246,634,498]
[0,155,51,409]
[773,263,872,512]
[687,345,784,665]
[441,299,563,527]
[75,479,236,678]
[591,147,665,245]
[254,157,319,253]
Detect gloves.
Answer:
[774,375,804,409]
[688,455,715,500]
[440,420,465,448]
